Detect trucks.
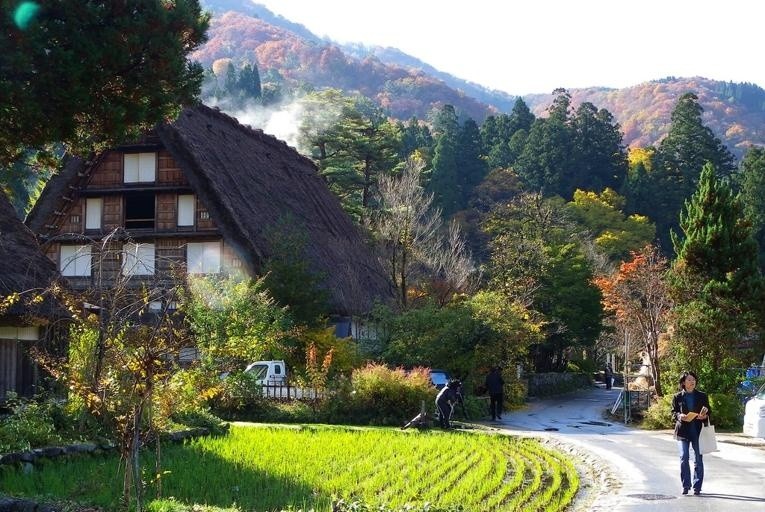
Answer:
[240,359,325,400]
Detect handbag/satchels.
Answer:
[699,416,720,455]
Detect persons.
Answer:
[671,371,711,497]
[434,379,462,430]
[603,363,613,392]
[484,365,505,421]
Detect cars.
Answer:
[732,366,761,407]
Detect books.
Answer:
[685,412,699,423]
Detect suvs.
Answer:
[403,368,455,392]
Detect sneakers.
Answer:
[683,488,688,494]
[694,488,699,495]
